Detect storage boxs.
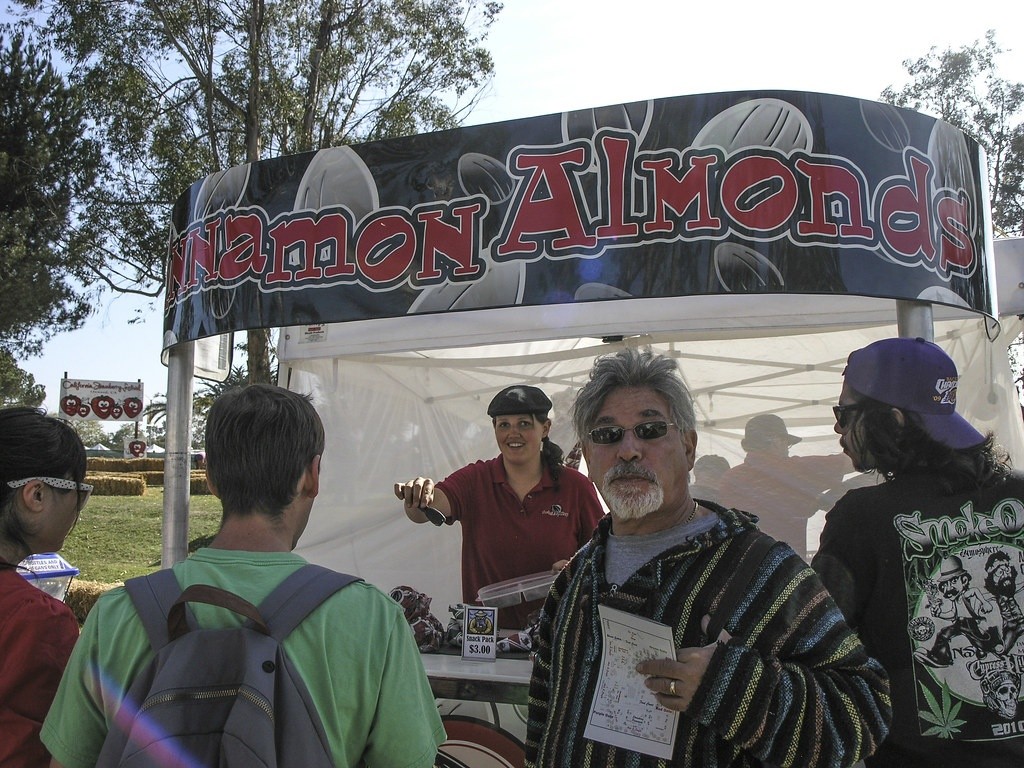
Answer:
[475,570,562,608]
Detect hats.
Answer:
[487,385,552,415]
[741,413,802,452]
[842,337,986,450]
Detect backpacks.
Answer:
[95,564,366,768]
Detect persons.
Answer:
[813,339,1024,768]
[0,405,94,768]
[394,385,606,640]
[41,385,449,768]
[523,350,893,768]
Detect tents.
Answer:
[156,90,1024,618]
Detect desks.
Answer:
[420,653,534,705]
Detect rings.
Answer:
[669,680,676,695]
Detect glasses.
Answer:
[7,476,94,511]
[586,420,674,446]
[784,437,793,449]
[833,404,863,428]
